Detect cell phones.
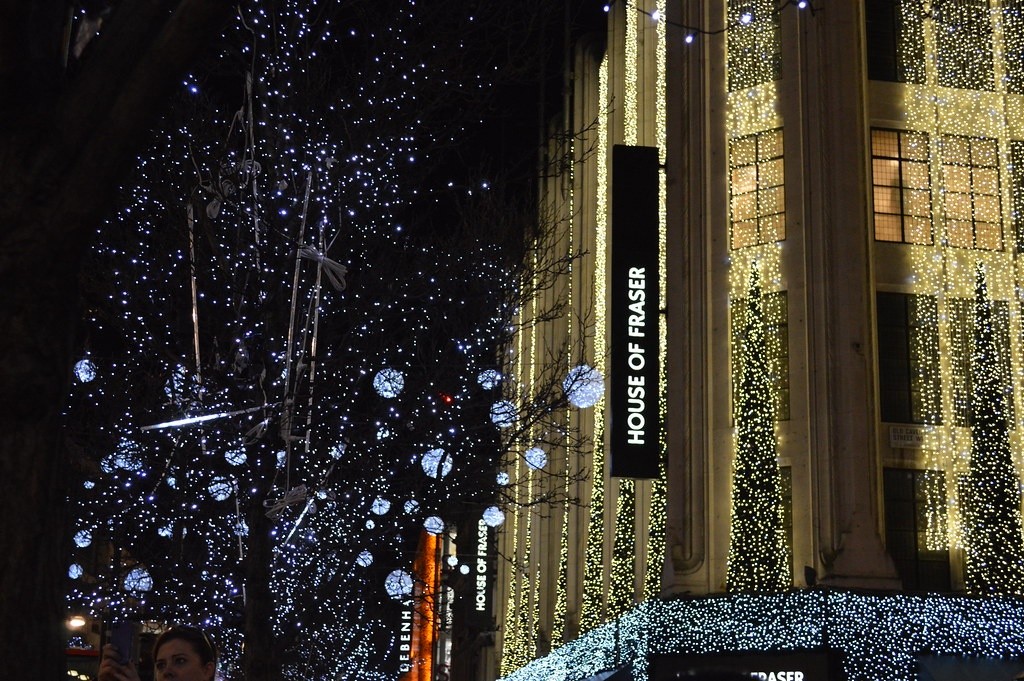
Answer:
[111,621,133,666]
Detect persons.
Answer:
[98,625,217,681]
